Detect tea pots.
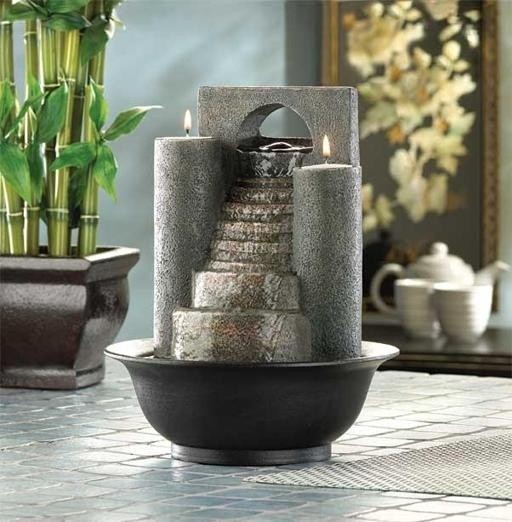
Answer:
[370,241,511,343]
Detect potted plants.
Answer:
[3,0,142,395]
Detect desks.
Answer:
[365,321,512,376]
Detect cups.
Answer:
[432,278,495,346]
[394,277,441,339]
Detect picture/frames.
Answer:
[318,0,499,317]
